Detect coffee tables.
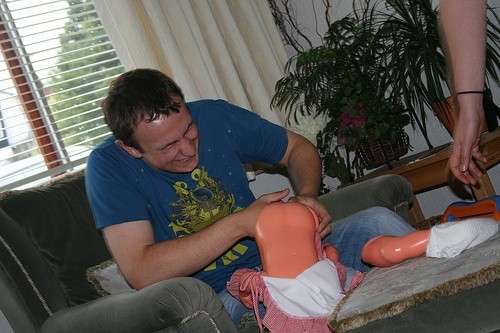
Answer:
[328,219,500,333]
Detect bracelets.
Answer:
[453,88,488,97]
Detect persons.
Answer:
[439,1,488,187]
[86,68,334,292]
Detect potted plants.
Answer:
[376,0,500,139]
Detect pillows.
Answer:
[86,258,134,297]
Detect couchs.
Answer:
[0,174,413,333]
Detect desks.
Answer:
[336,128,500,225]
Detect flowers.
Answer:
[269,19,415,187]
